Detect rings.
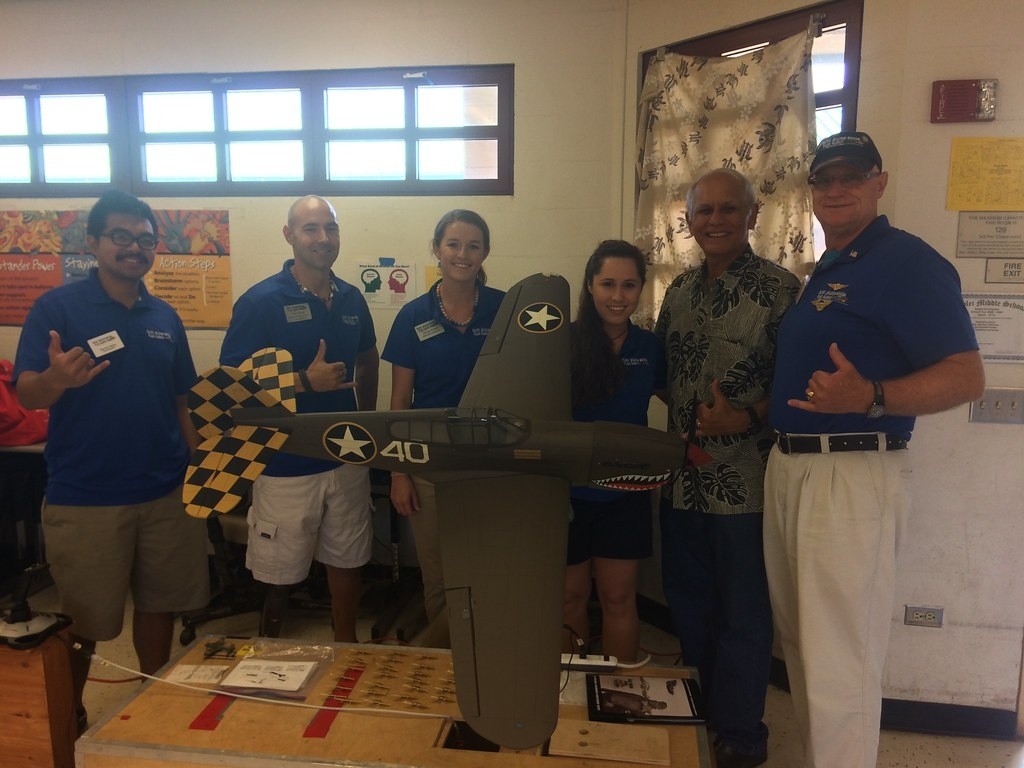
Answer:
[87,364,91,370]
[340,369,345,376]
[696,419,701,428]
[805,392,814,401]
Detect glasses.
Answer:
[807,172,879,190]
[93,232,157,251]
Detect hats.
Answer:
[806,131,882,184]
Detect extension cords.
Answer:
[558,653,618,673]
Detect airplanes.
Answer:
[181,271,690,749]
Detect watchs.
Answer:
[865,379,887,420]
[741,407,762,436]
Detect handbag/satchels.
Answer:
[0,359,49,446]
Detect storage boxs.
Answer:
[74,633,712,768]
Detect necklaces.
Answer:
[608,329,628,345]
[435,281,480,326]
[290,269,335,301]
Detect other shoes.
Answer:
[710,737,768,767]
[73,706,87,733]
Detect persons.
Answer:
[378,208,509,650]
[220,195,378,648]
[649,169,802,768]
[9,196,209,736]
[561,239,671,667]
[758,130,984,768]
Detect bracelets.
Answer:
[391,473,407,477]
[298,369,315,393]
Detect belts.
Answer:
[770,430,907,455]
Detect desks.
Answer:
[0,445,422,639]
